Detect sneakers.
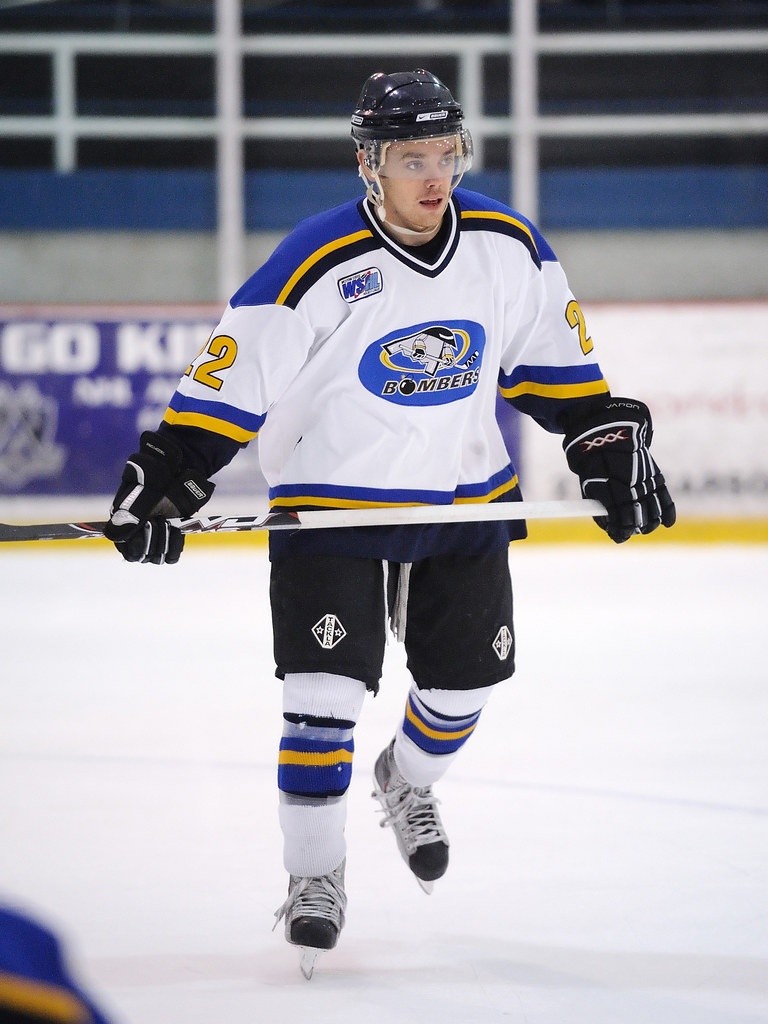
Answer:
[370,736,450,895]
[271,856,349,979]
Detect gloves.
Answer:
[561,397,677,545]
[100,428,217,566]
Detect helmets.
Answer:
[349,67,466,141]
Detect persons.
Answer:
[103,69,677,950]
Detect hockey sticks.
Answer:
[0,492,624,525]
